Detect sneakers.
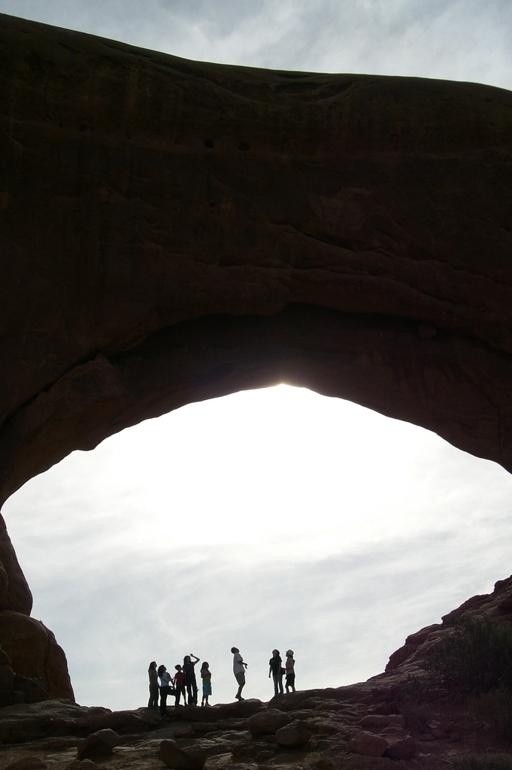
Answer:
[202,702,210,706]
[235,696,245,701]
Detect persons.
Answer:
[149,661,159,708]
[268,648,283,695]
[230,646,248,702]
[183,653,200,707]
[173,664,187,705]
[200,661,212,707]
[282,649,295,693]
[157,665,177,707]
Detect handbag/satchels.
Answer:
[167,686,176,696]
[281,667,285,674]
